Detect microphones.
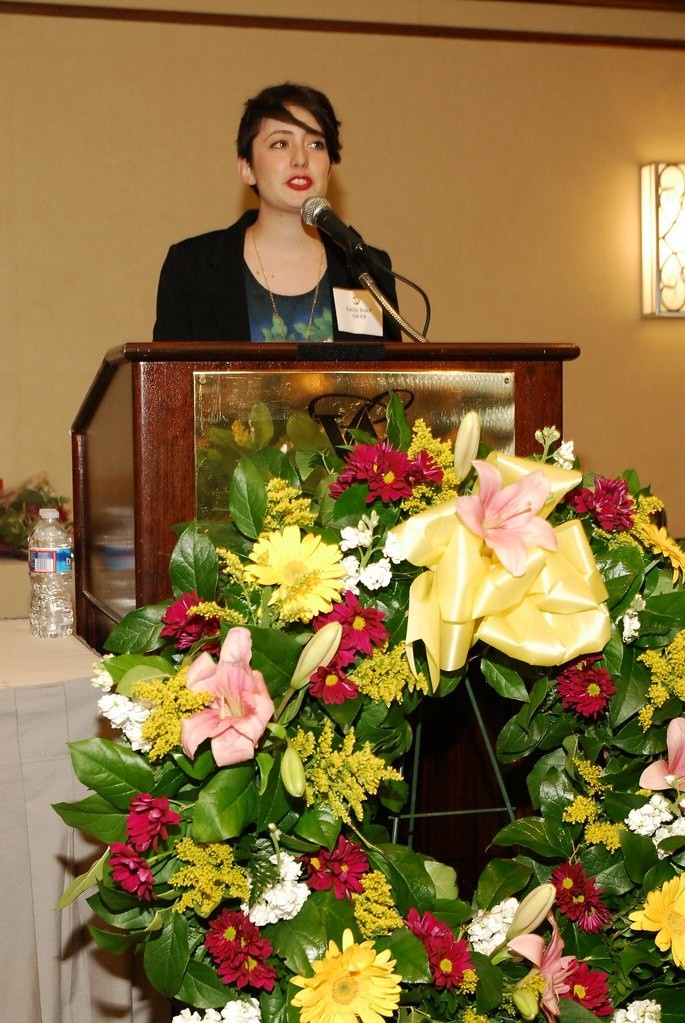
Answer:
[300,197,382,270]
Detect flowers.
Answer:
[58,380,685,1023]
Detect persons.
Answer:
[152,82,402,343]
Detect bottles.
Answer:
[28,508,75,639]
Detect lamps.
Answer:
[640,162,685,318]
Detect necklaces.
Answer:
[252,225,323,341]
